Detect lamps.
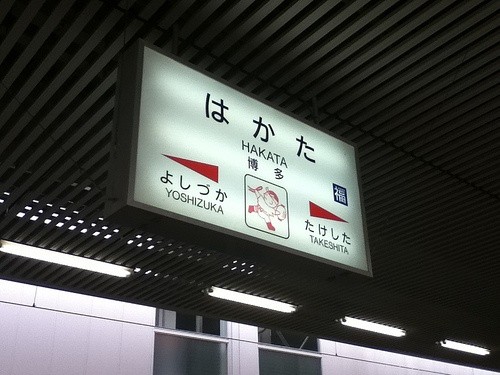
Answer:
[207,286,297,314]
[0,240,132,278]
[439,338,491,356]
[335,316,407,338]
